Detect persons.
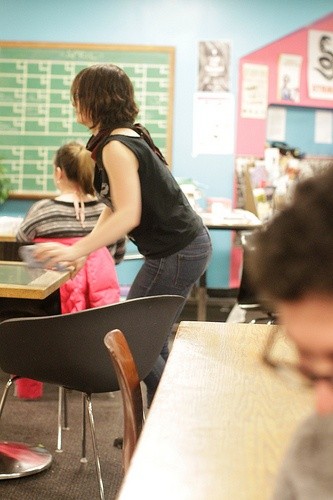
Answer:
[16,63,213,450]
[249,163,333,500]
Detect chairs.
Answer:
[103,329,156,484]
[0,292,190,500]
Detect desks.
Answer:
[117,321,320,500]
[178,211,262,324]
[0,215,90,480]
